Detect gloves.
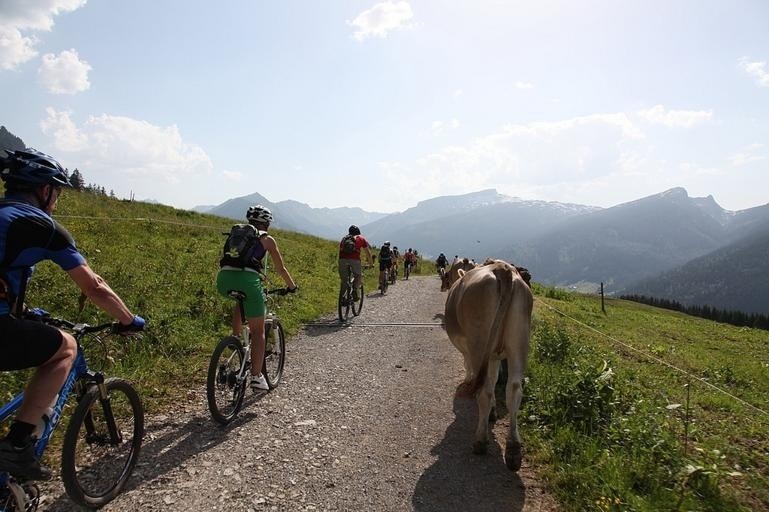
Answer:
[284,285,300,294]
[27,306,50,317]
[118,316,146,329]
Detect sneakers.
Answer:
[1,434,52,481]
[249,374,271,390]
[353,287,359,302]
[228,333,242,350]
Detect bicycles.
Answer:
[206,284,297,425]
[337,263,374,320]
[0,308,152,512]
[380,262,412,294]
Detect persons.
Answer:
[378,240,420,295]
[0,145,145,486]
[216,204,298,394]
[339,224,375,302]
[434,251,475,276]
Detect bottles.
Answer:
[32,391,59,443]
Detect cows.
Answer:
[439,257,477,292]
[493,264,531,390]
[443,260,533,473]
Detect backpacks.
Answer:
[343,235,355,253]
[381,246,389,258]
[392,249,398,256]
[223,223,267,266]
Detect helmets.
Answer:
[2,146,73,189]
[348,225,360,235]
[246,204,274,223]
[384,240,390,245]
